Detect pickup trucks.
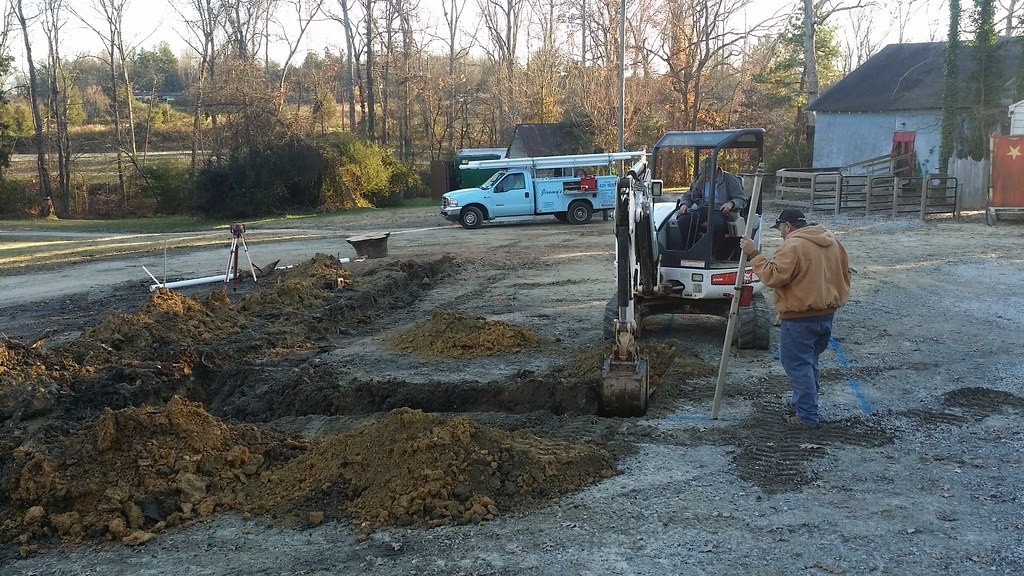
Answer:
[441,166,618,229]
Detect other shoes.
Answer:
[781,415,821,428]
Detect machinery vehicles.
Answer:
[600,126,774,416]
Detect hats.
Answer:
[770,208,806,229]
[700,157,716,172]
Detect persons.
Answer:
[740,209,851,427]
[677,157,747,256]
[504,175,524,191]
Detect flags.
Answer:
[991,135,1024,208]
